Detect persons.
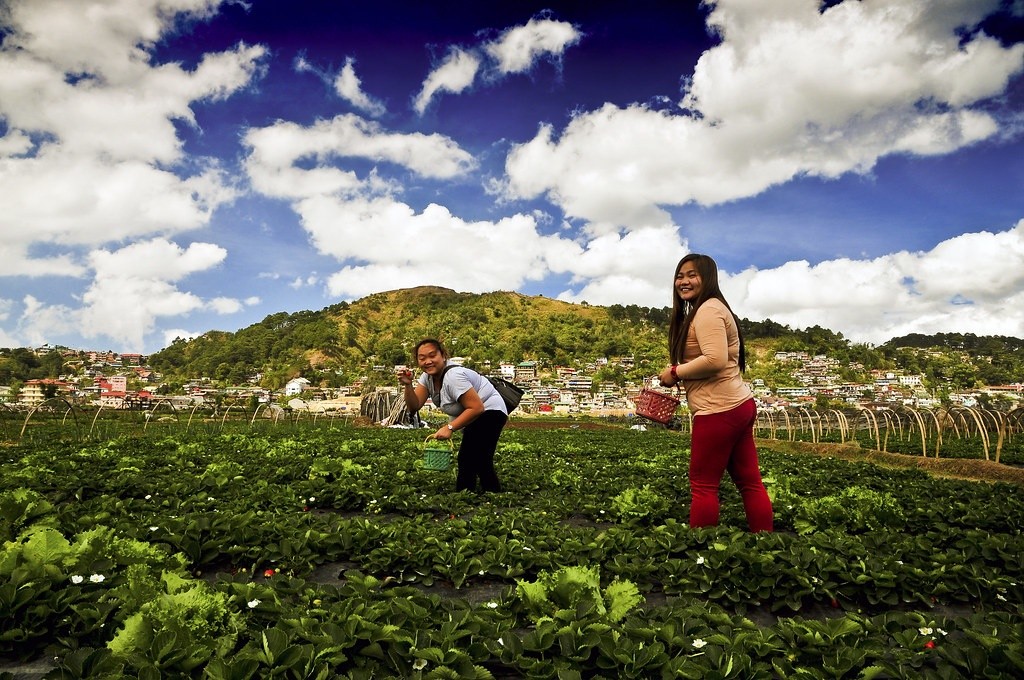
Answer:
[657,254,774,533]
[396,338,509,494]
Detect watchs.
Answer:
[671,365,682,382]
[447,423,456,432]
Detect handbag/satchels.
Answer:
[486,376,524,414]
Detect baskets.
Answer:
[635,374,681,424]
[421,434,454,471]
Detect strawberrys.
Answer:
[0,431,1024,679]
